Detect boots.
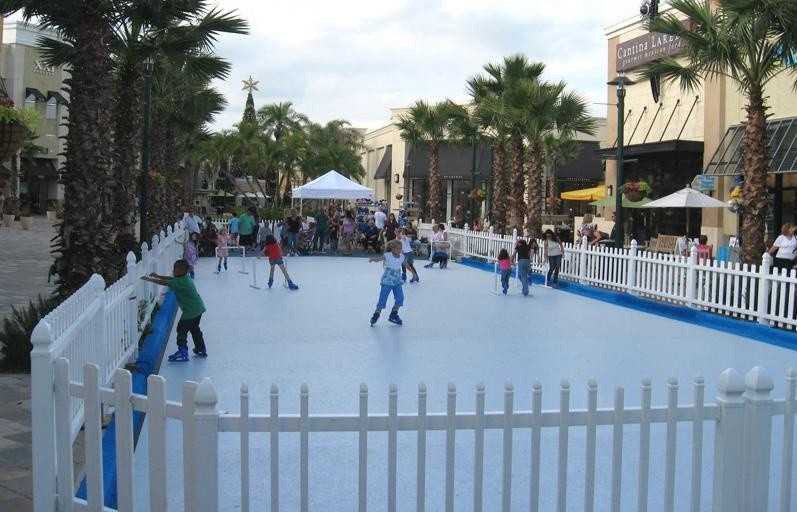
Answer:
[387,310,402,325]
[409,272,419,283]
[423,262,433,269]
[268,277,274,288]
[190,272,195,279]
[400,272,407,282]
[190,345,208,357]
[287,278,299,290]
[368,310,380,325]
[439,264,445,269]
[166,345,189,360]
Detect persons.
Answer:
[768,222,797,278]
[141,260,206,358]
[369,240,417,324]
[498,249,512,294]
[174,205,447,289]
[542,229,565,288]
[527,239,541,284]
[511,240,531,296]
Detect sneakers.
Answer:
[546,280,550,286]
[501,286,529,297]
[224,262,228,270]
[552,279,558,289]
[218,264,221,272]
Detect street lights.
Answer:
[138,52,156,247]
[606,68,636,248]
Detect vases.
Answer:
[630,195,645,203]
[0,125,28,161]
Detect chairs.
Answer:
[196,224,457,264]
[541,216,616,246]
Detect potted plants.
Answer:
[2,197,59,231]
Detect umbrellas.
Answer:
[560,183,733,256]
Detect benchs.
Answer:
[645,234,712,260]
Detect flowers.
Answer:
[546,196,562,207]
[617,182,653,195]
[0,91,43,136]
[471,187,488,200]
